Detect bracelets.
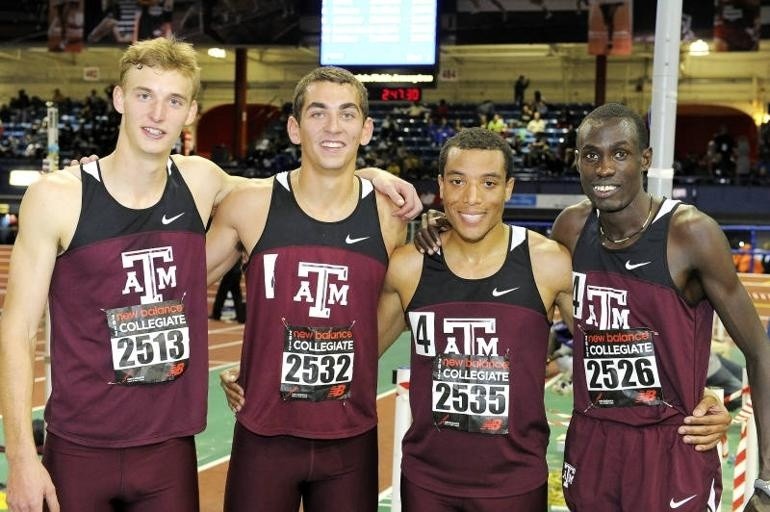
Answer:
[755,476,770,497]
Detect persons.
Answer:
[2,84,123,243]
[355,99,580,180]
[63,64,410,512]
[749,121,770,186]
[413,99,770,511]
[208,256,247,324]
[761,240,770,274]
[218,123,733,511]
[580,103,593,118]
[513,75,531,106]
[673,126,739,187]
[210,102,302,179]
[0,32,426,512]
[173,128,195,156]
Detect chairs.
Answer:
[0,107,119,166]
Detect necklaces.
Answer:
[593,197,653,244]
